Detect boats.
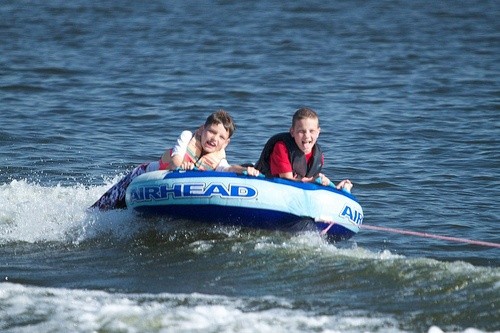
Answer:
[125,168,364,241]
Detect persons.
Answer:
[88,111,259,212]
[256,106,353,193]
